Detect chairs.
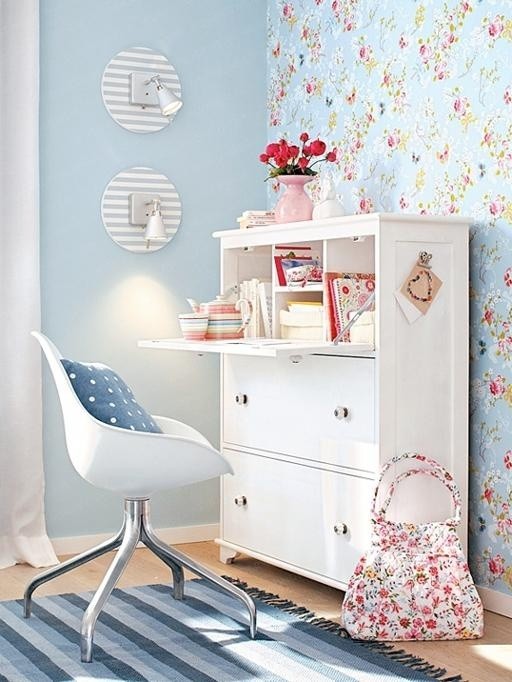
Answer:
[22,330,258,663]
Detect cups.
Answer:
[179,314,209,341]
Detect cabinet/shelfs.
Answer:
[138,213,474,592]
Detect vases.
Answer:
[275,175,315,224]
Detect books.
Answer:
[237,244,376,344]
[236,209,276,229]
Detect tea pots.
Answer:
[185,294,252,340]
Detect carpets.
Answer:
[0,574,467,682]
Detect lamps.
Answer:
[129,72,183,116]
[130,192,170,240]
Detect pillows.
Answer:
[59,358,163,434]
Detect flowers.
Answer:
[259,133,336,182]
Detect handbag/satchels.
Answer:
[341,517,485,643]
